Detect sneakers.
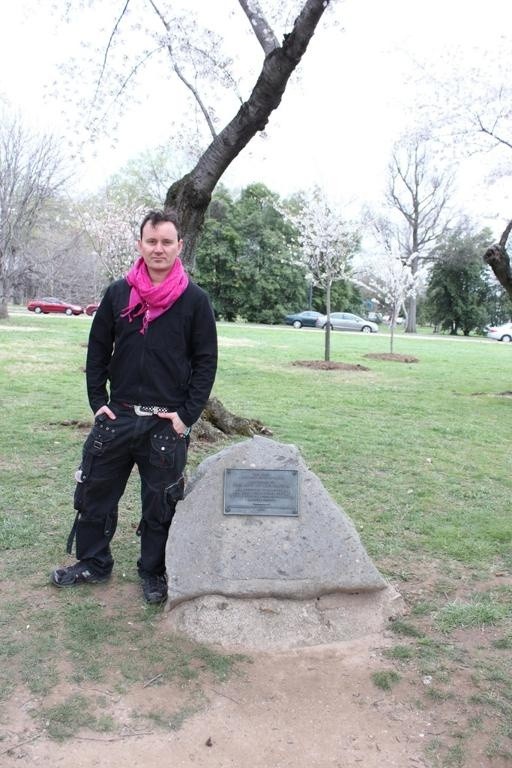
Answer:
[51,561,110,587]
[141,575,167,603]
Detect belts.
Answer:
[128,403,166,417]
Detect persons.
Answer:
[47,206,218,607]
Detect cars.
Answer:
[27,297,85,315]
[284,310,324,328]
[86,303,99,316]
[316,311,380,334]
[487,322,512,342]
[368,311,404,326]
